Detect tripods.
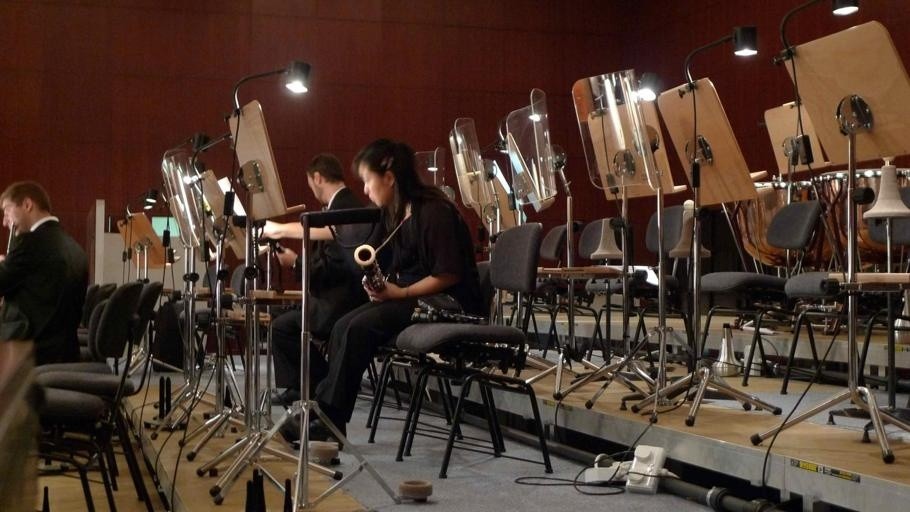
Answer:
[751,127,909,463]
[554,164,656,403]
[209,216,404,511]
[126,246,186,379]
[198,214,342,480]
[151,240,223,440]
[113,243,168,370]
[178,214,273,461]
[630,161,781,425]
[585,170,711,410]
[523,196,617,399]
[724,155,822,332]
[468,207,579,380]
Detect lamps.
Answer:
[679,26,760,184]
[128,181,154,219]
[137,181,175,253]
[495,108,551,205]
[778,0,861,161]
[634,70,664,103]
[211,59,325,226]
[160,133,214,248]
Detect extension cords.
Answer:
[585,463,629,485]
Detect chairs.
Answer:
[700,199,824,386]
[77,284,100,327]
[41,300,122,464]
[592,204,694,372]
[36,281,143,510]
[394,221,553,477]
[16,281,163,491]
[77,279,116,334]
[526,219,630,360]
[521,224,573,356]
[365,261,505,455]
[781,199,910,404]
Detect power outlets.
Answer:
[583,460,632,486]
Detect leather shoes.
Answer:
[292,429,347,450]
[271,388,309,404]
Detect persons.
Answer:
[281,140,484,454]
[260,154,370,409]
[2,178,90,367]
